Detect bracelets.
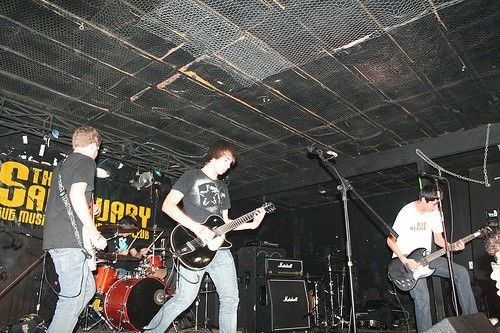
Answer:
[402,257,407,263]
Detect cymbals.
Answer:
[143,227,167,232]
[301,276,324,280]
[95,252,140,263]
[314,256,344,263]
[333,269,346,274]
[97,223,140,233]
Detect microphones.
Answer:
[308,147,338,158]
[302,313,310,319]
[160,295,168,299]
[420,171,445,179]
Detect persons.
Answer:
[484,228,500,296]
[143,143,266,333]
[102,215,149,275]
[387,184,498,333]
[40,126,107,333]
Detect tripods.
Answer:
[316,250,350,327]
[79,305,116,333]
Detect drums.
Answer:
[101,273,173,333]
[91,264,120,298]
[306,281,324,314]
[131,254,167,279]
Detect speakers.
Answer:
[237,246,312,333]
[423,313,499,333]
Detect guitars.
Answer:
[170,202,276,271]
[82,193,97,271]
[387,226,493,293]
